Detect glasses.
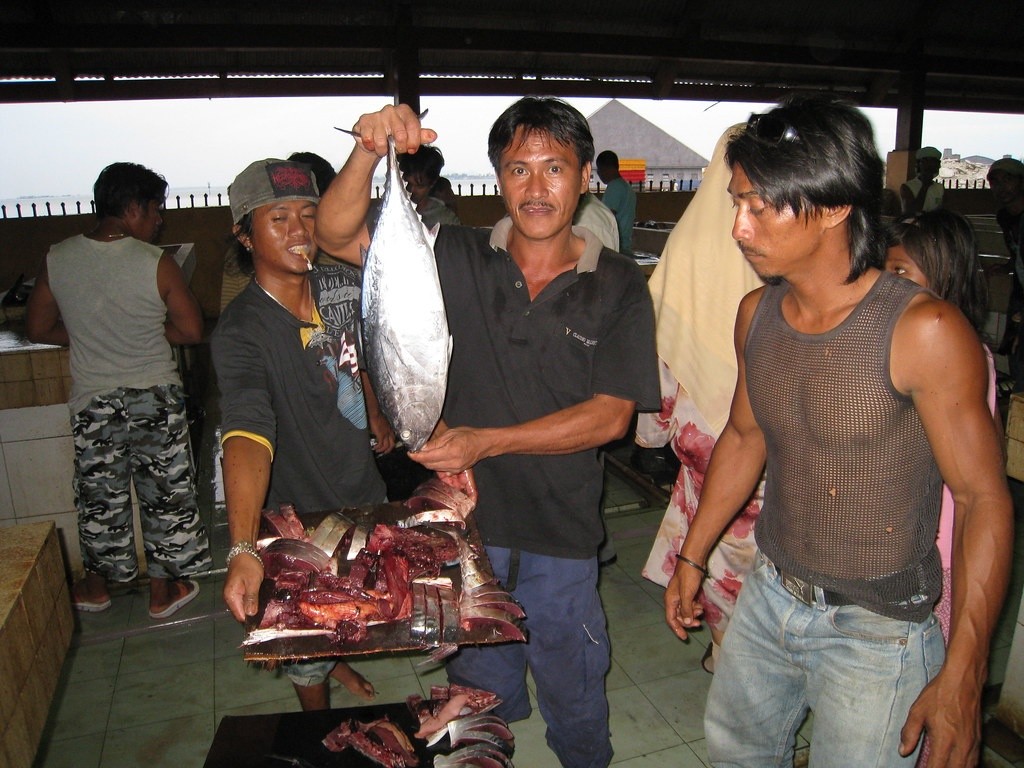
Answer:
[746,113,808,151]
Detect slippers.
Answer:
[71,578,111,612]
[148,580,200,618]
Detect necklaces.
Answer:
[104,232,124,239]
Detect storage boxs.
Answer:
[1003,392,1024,481]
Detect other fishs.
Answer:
[334,106,457,452]
[250,477,530,768]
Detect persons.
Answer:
[572,190,619,565]
[365,144,461,502]
[212,158,395,711]
[664,88,1013,767]
[26,162,212,619]
[221,152,337,314]
[596,150,634,256]
[880,147,1024,766]
[635,124,768,675]
[313,97,663,768]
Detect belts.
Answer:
[774,545,937,607]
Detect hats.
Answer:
[987,158,1024,181]
[229,158,320,225]
[916,146,942,164]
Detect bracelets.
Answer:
[225,541,265,569]
[675,554,706,574]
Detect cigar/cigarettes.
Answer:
[300,249,312,271]
